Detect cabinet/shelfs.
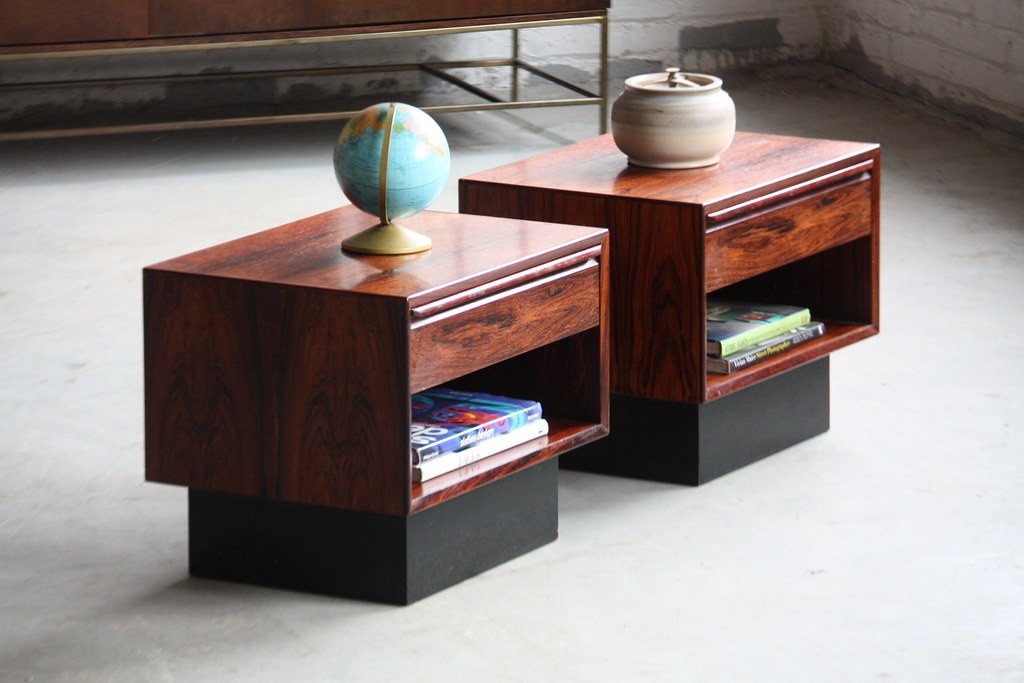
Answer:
[143,200,612,605]
[457,129,880,487]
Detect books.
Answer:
[705,296,825,375]
[410,386,549,482]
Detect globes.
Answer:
[335,101,452,256]
